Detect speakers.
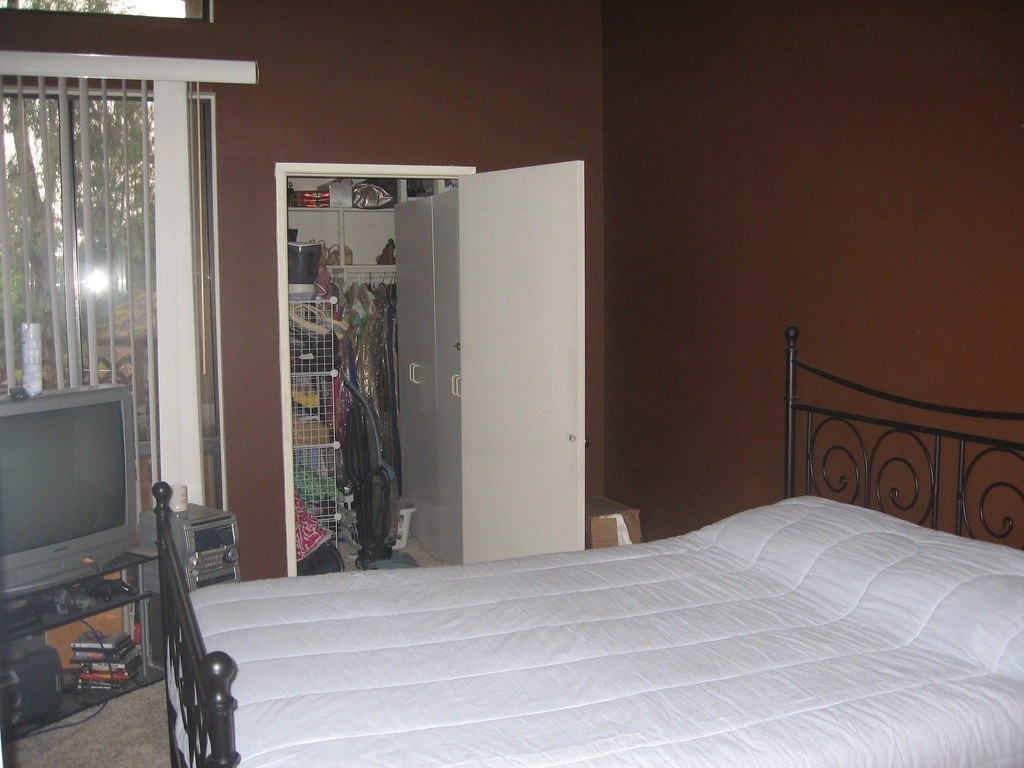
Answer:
[0,636,65,730]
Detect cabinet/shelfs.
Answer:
[397,186,458,565]
[287,174,460,577]
[0,548,166,743]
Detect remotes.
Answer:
[9,387,28,399]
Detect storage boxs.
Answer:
[585,494,642,550]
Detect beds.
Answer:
[156,325,1024,768]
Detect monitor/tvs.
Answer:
[0,382,138,600]
